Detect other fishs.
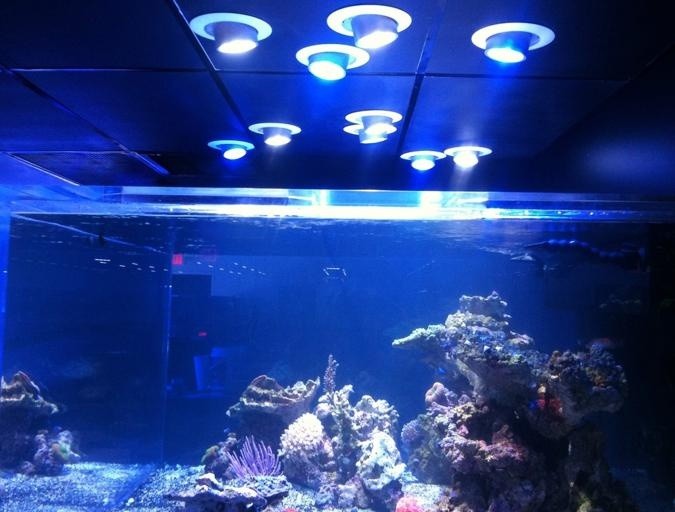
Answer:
[524,238,624,261]
[509,253,537,262]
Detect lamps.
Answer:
[342,109,403,144]
[399,145,492,172]
[207,139,255,161]
[247,122,302,146]
[470,21,556,64]
[188,11,273,55]
[295,4,413,81]
